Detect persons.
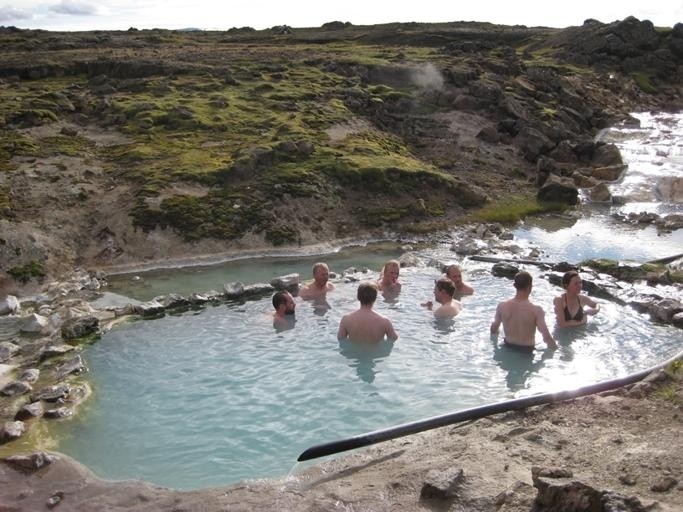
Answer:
[271,292,296,322]
[447,263,474,295]
[297,262,335,297]
[490,271,557,351]
[553,270,600,327]
[336,281,398,342]
[421,277,463,319]
[376,259,402,291]
